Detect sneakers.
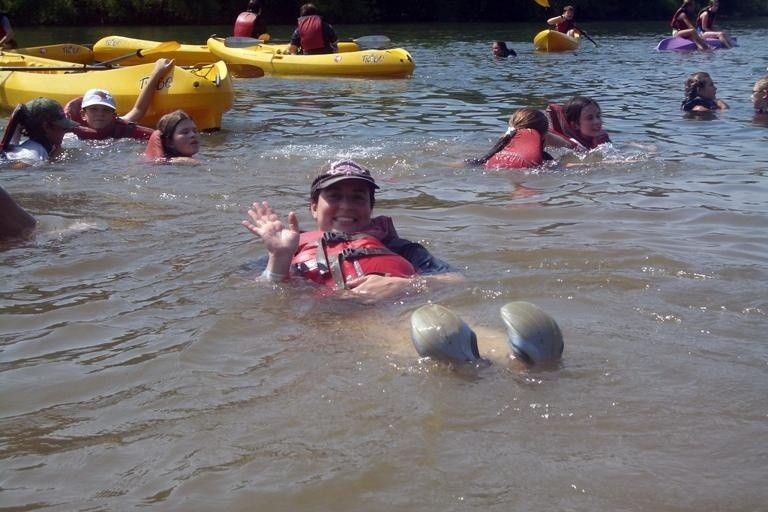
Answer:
[410,303,482,372]
[500,301,565,367]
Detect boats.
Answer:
[533,29,581,51]
[4,42,95,63]
[656,36,737,50]
[93,35,359,67]
[207,35,416,77]
[0,52,237,132]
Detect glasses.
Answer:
[751,89,762,94]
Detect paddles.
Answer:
[536,1,598,49]
[224,35,391,50]
[94,41,181,66]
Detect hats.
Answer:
[81,88,117,110]
[20,96,81,130]
[310,159,380,198]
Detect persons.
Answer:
[289,3,339,55]
[0,186,36,240]
[233,0,267,39]
[155,109,202,167]
[547,6,577,38]
[750,77,768,114]
[492,40,517,59]
[79,57,176,129]
[446,96,613,172]
[241,158,564,368]
[670,0,734,51]
[0,97,81,170]
[684,72,729,113]
